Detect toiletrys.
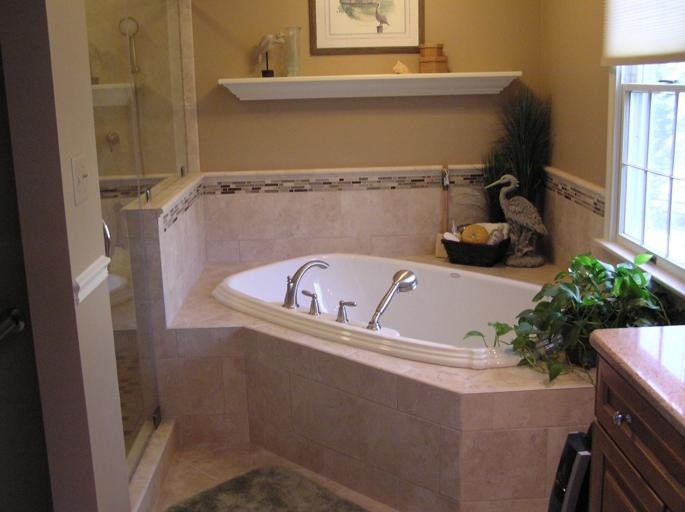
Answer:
[487,224,505,247]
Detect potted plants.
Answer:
[463,251,673,384]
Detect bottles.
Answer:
[283,26,302,77]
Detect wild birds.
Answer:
[484,173,549,260]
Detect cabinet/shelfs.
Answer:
[589,351,684,512]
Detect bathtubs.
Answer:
[214,252,568,369]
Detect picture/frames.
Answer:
[308,0,426,55]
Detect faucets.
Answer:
[283,259,330,309]
[368,269,418,328]
